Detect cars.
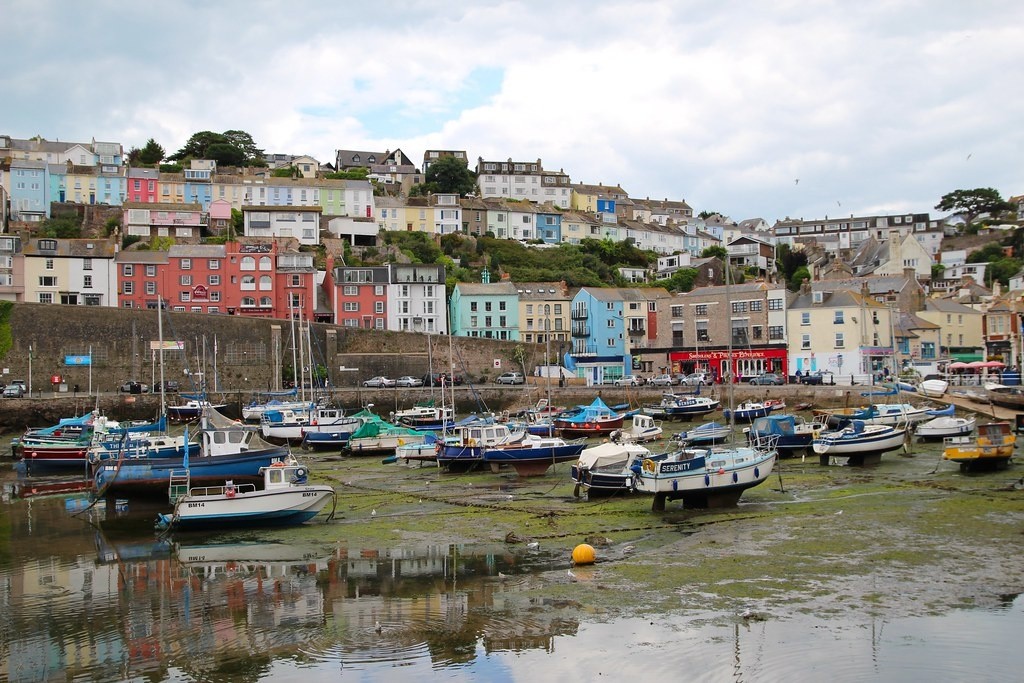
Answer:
[120,380,148,393]
[363,376,396,388]
[396,376,423,388]
[496,370,524,385]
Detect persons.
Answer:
[724,371,730,385]
[814,368,824,385]
[712,371,717,382]
[804,369,810,376]
[18,385,24,398]
[266,378,273,392]
[441,374,448,388]
[795,368,802,384]
[737,373,742,385]
[351,378,356,389]
[872,360,912,387]
[283,380,301,401]
[453,374,462,385]
[703,375,708,385]
[325,378,329,390]
[560,373,566,387]
[938,362,1020,382]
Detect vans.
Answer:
[155,380,179,392]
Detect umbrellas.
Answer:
[983,362,1005,368]
[946,362,968,370]
[969,361,985,369]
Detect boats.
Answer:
[0,290,1016,532]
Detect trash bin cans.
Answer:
[73,385,79,392]
[559,379,563,387]
[130,384,141,394]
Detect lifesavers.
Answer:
[812,430,821,441]
[273,462,287,466]
[468,439,476,447]
[643,458,655,472]
